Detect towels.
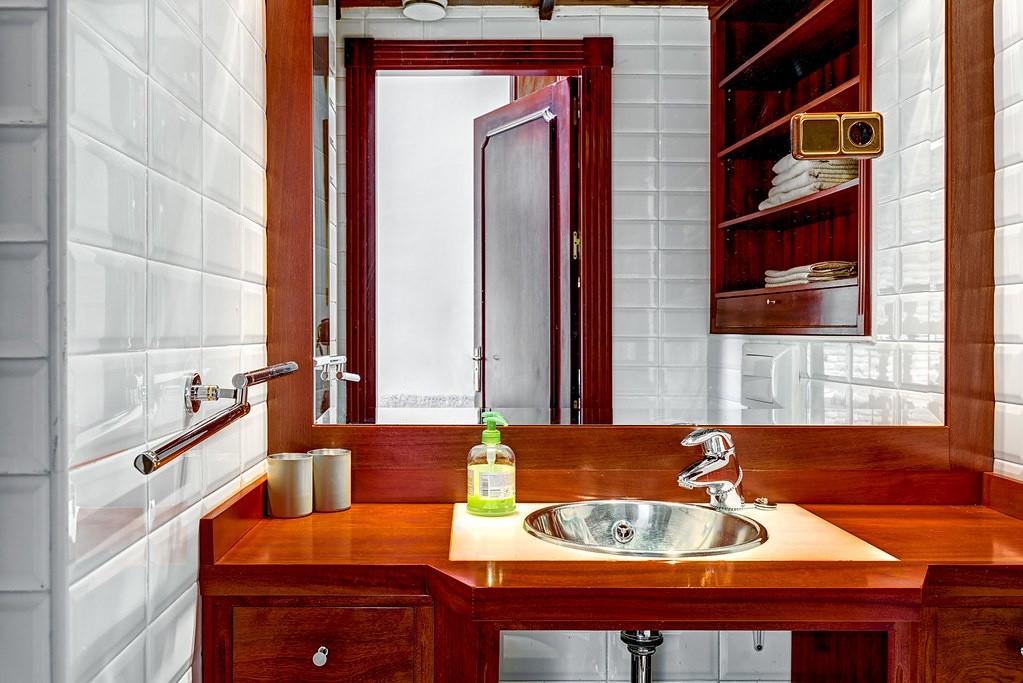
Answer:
[765,261,858,288]
[758,153,858,211]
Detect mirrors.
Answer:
[263,0,998,505]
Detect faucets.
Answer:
[676,428,745,509]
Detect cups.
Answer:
[266,452,313,518]
[307,448,356,511]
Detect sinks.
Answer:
[523,500,767,557]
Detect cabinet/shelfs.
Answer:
[195,471,1023,683]
[705,0,872,336]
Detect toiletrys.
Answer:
[467,417,516,517]
[481,412,503,417]
